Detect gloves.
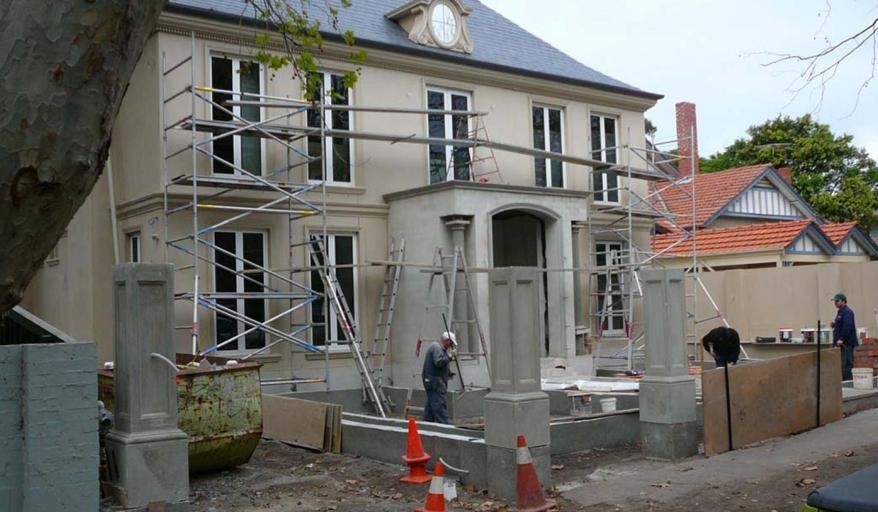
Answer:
[449,348,458,360]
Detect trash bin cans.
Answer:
[97,353,265,477]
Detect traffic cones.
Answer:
[507,435,557,512]
[399,415,434,483]
[415,462,446,512]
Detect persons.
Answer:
[703,326,740,366]
[830,294,858,382]
[422,330,458,424]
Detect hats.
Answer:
[830,293,846,301]
[442,331,458,346]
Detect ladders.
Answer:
[444,113,502,183]
[593,248,635,377]
[304,233,492,419]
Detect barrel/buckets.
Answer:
[855,327,869,344]
[852,369,873,389]
[569,395,591,419]
[599,396,617,412]
